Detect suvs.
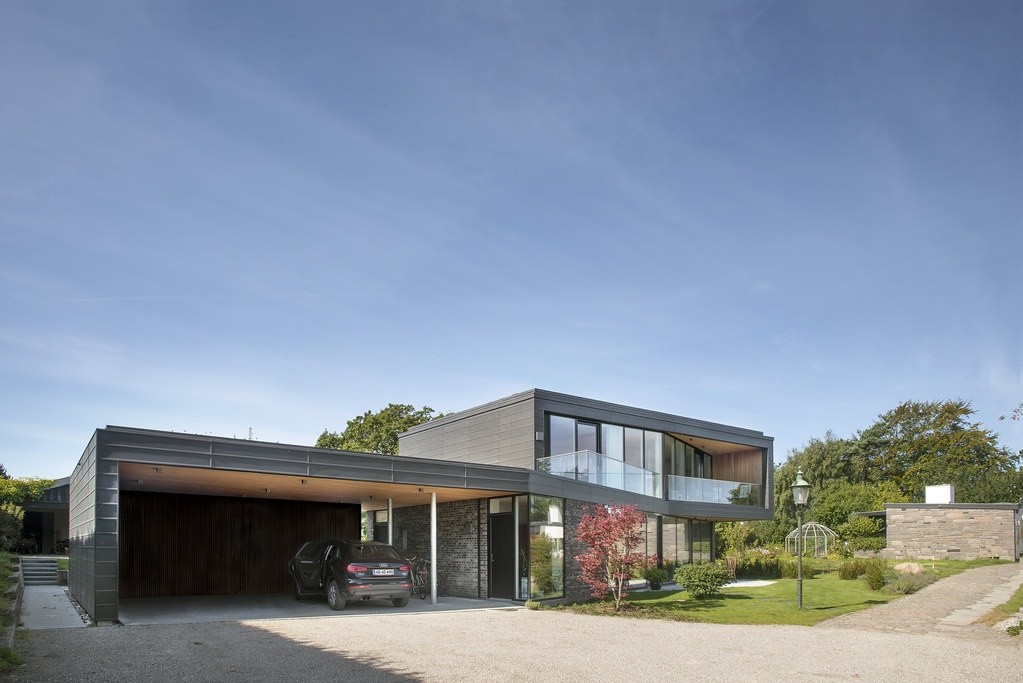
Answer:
[288,538,412,611]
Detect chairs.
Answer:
[735,485,751,505]
[725,556,737,583]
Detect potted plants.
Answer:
[56,560,68,586]
[644,569,669,590]
[521,568,533,598]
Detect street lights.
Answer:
[789,465,812,610]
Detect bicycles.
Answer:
[54,538,69,555]
[404,556,443,600]
